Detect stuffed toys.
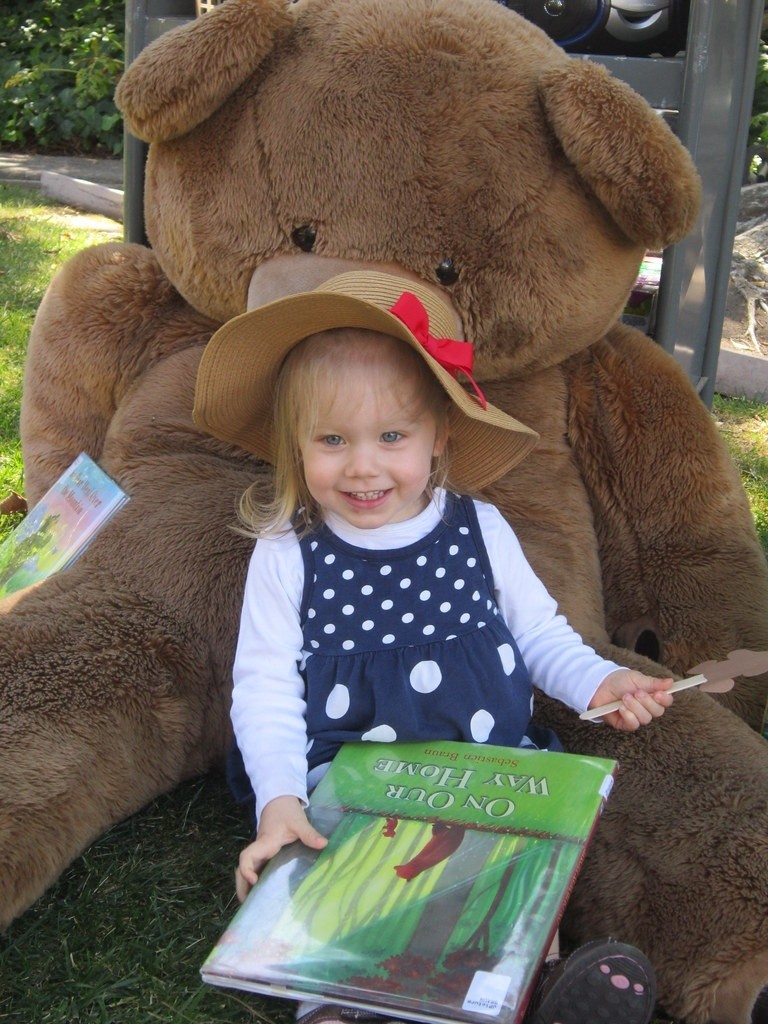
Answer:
[0,0,768,1024]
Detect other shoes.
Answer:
[295,1005,405,1024]
[521,939,655,1024]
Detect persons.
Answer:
[191,273,675,1024]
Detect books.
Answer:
[200,741,620,1024]
[0,451,129,617]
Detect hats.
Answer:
[191,270,541,497]
